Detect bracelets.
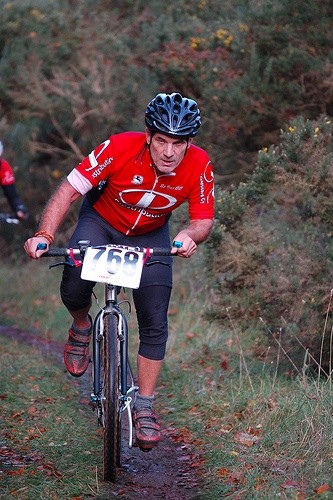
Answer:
[34,231,55,245]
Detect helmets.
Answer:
[144,91,202,138]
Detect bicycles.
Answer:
[34,239,183,484]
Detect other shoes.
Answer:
[132,404,161,447]
[64,314,93,376]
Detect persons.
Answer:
[0,141,28,221]
[24,93,216,449]
